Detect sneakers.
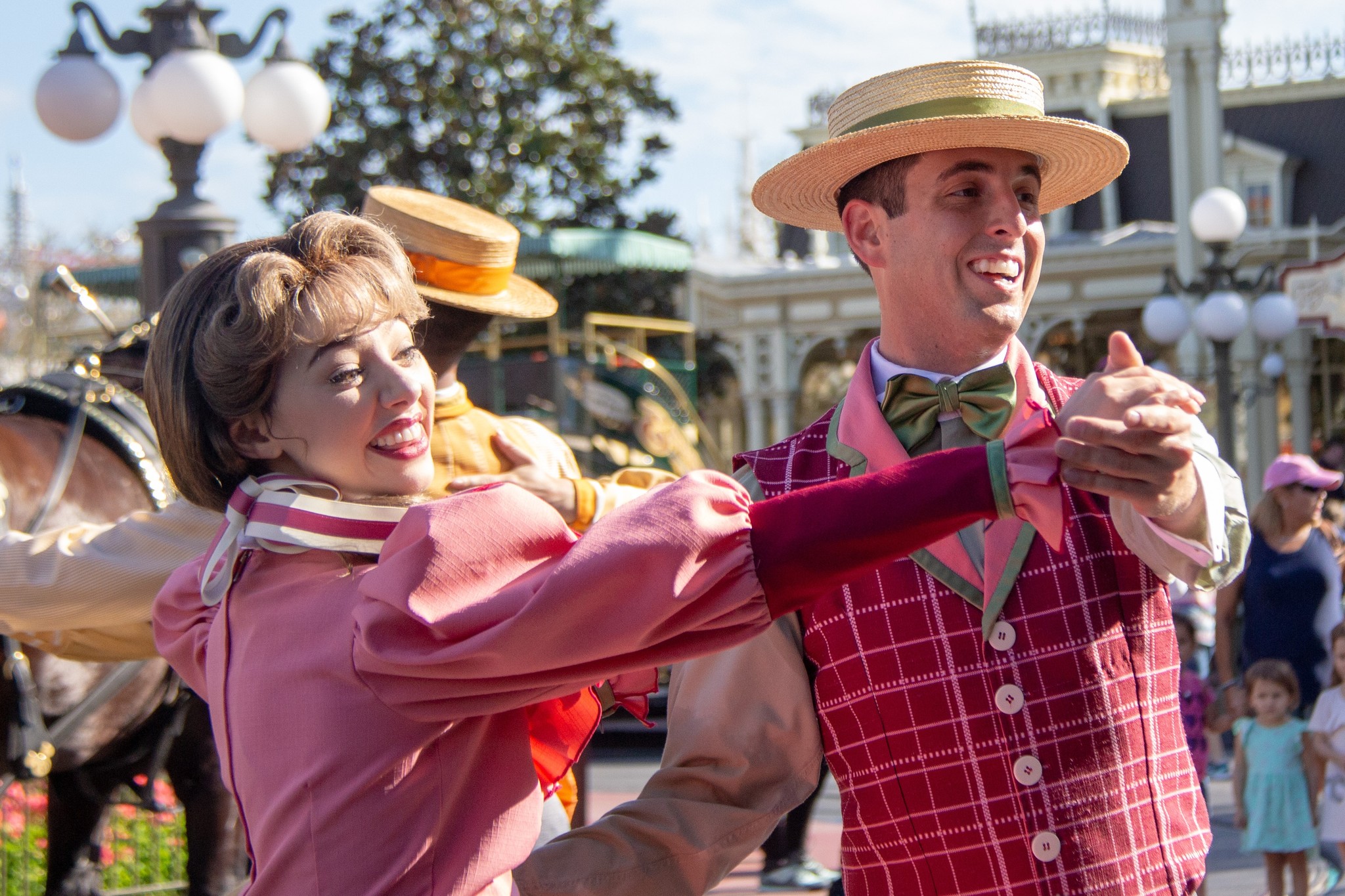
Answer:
[760,855,842,891]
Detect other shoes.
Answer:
[1305,867,1340,896]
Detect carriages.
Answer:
[1,218,699,896]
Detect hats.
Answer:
[1262,451,1344,493]
[360,186,559,319]
[751,60,1131,233]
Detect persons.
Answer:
[1229,660,1318,896]
[1307,623,1345,868]
[1170,610,1243,896]
[756,756,843,890]
[2,186,680,845]
[1166,426,1345,782]
[143,213,1209,896]
[511,62,1249,896]
[1215,453,1345,712]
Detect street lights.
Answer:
[34,0,334,339]
[1141,186,1298,478]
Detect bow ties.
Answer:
[880,362,1017,453]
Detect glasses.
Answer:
[1293,484,1322,493]
